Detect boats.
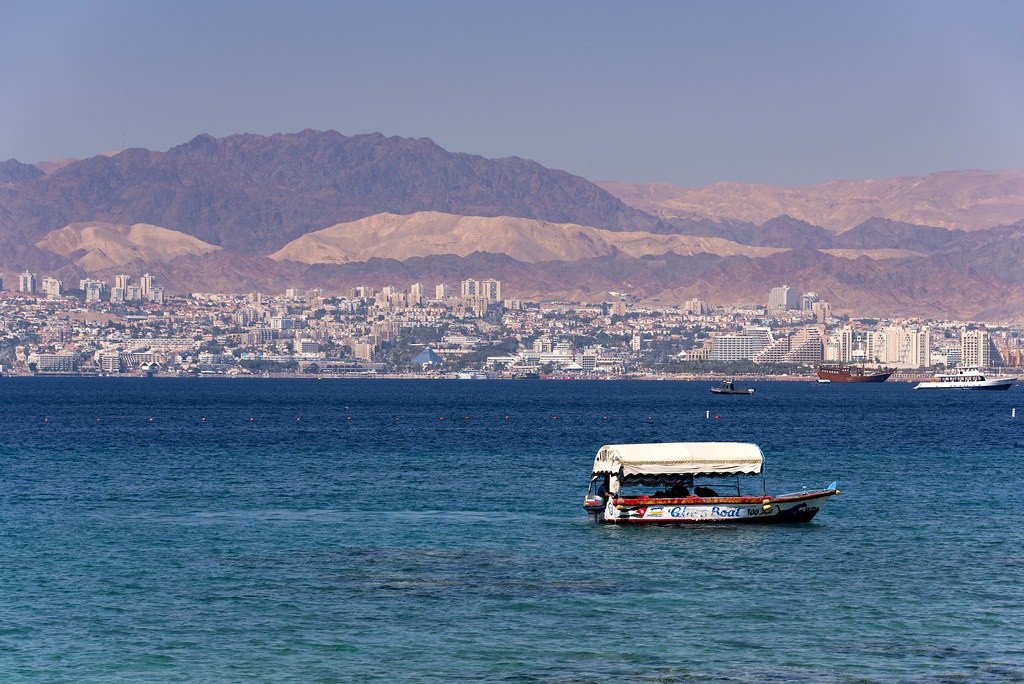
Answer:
[583,442,840,524]
[709,379,756,394]
[816,346,892,382]
[816,379,830,382]
[913,366,1018,391]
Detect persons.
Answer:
[652,485,719,499]
[598,477,614,504]
